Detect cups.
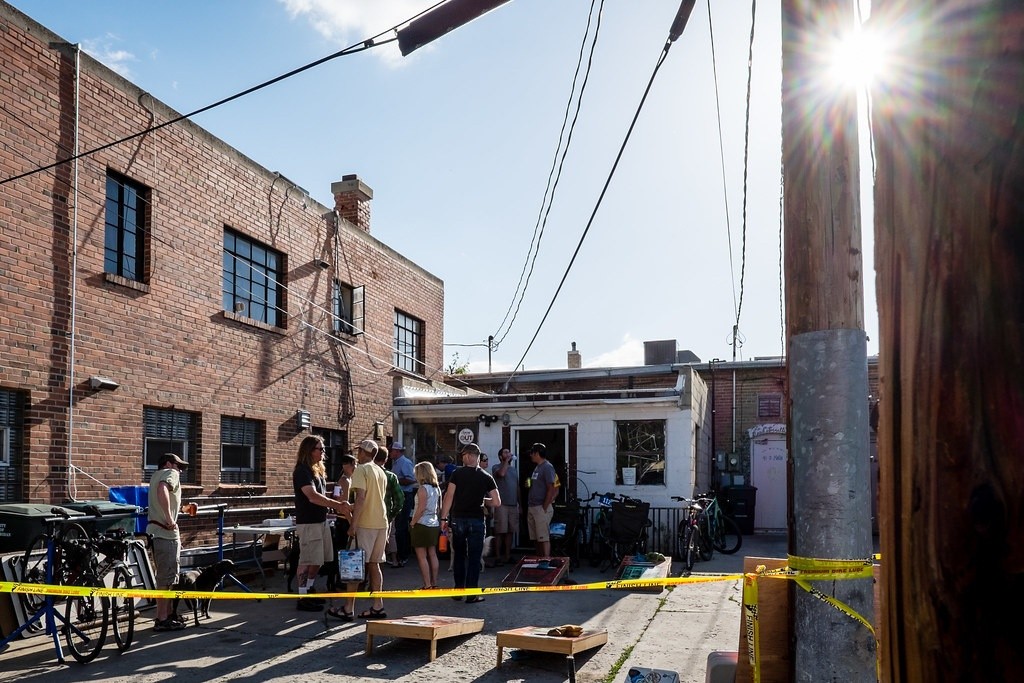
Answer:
[333,485,342,497]
[189,503,198,516]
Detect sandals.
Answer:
[328,606,354,622]
[358,607,387,618]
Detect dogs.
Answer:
[443,524,495,573]
[171,558,238,628]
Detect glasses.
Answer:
[482,458,488,462]
[315,448,324,452]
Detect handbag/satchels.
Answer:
[338,533,365,581]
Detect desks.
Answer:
[218,515,337,593]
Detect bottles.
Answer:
[511,454,518,461]
[439,530,447,552]
[526,476,531,487]
[279,509,284,519]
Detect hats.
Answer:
[459,443,480,455]
[388,442,403,450]
[526,443,546,452]
[354,440,378,455]
[158,453,189,472]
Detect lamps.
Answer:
[318,259,330,270]
[90,375,120,391]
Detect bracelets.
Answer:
[441,518,448,521]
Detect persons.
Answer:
[330,454,359,592]
[526,470,561,504]
[328,440,389,619]
[441,442,502,604]
[480,453,489,469]
[492,449,519,565]
[410,461,442,589]
[388,442,416,567]
[373,447,406,521]
[527,443,556,559]
[294,436,351,612]
[148,455,191,629]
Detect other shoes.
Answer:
[419,586,432,589]
[390,561,400,568]
[168,610,185,623]
[503,556,518,564]
[153,618,186,632]
[297,598,324,612]
[465,596,485,603]
[452,596,463,601]
[495,559,505,567]
[308,586,320,604]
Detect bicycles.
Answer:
[19,503,155,665]
[671,485,743,571]
[547,489,646,574]
